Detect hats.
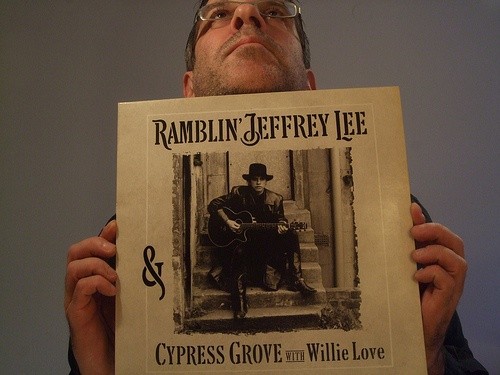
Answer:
[242,163,274,181]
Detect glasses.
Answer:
[191,1,302,48]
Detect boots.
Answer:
[288,252,318,296]
[232,272,248,320]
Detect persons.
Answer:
[208,163,322,318]
[63,0,490,375]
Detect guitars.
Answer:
[207,207,307,248]
